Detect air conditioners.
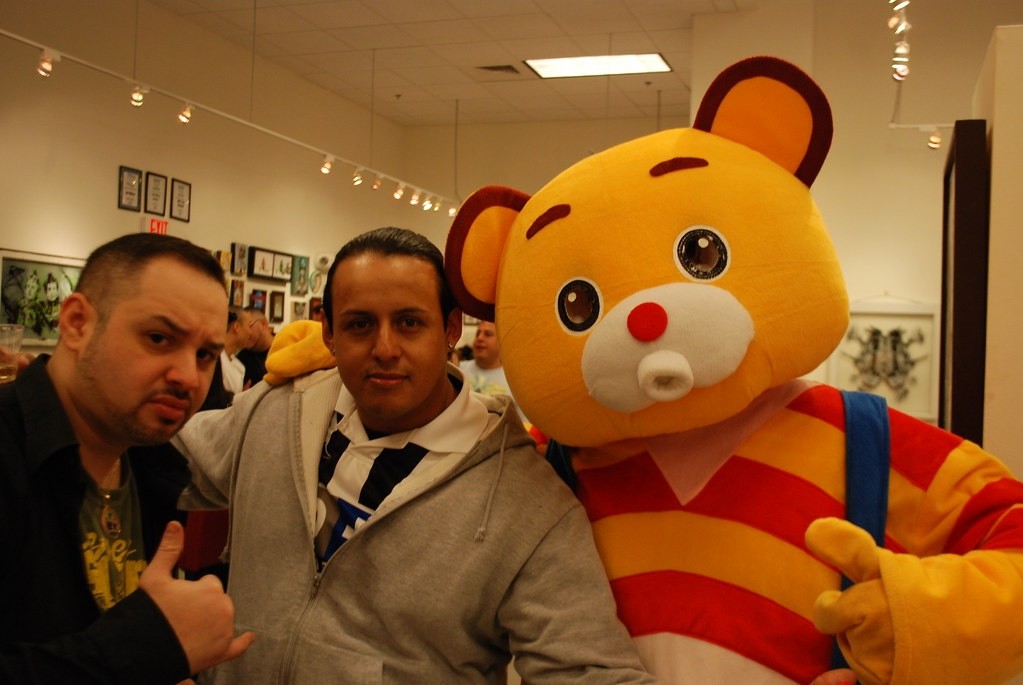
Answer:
[351,167,363,187]
[409,190,421,205]
[433,201,441,212]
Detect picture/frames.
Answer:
[118,166,143,212]
[0,248,88,347]
[143,172,168,216]
[463,312,481,325]
[169,178,191,223]
[824,310,940,423]
[197,242,333,324]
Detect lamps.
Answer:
[448,207,457,218]
[422,196,432,210]
[928,129,942,151]
[129,85,150,108]
[176,104,196,127]
[320,155,335,175]
[393,184,406,200]
[371,176,382,191]
[887,0,912,82]
[31,49,61,82]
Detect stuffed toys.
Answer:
[264,56,1023,684]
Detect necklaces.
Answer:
[76,427,122,542]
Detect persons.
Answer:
[0,232,257,685]
[310,304,322,323]
[458,320,533,434]
[169,228,657,684]
[199,305,275,412]
